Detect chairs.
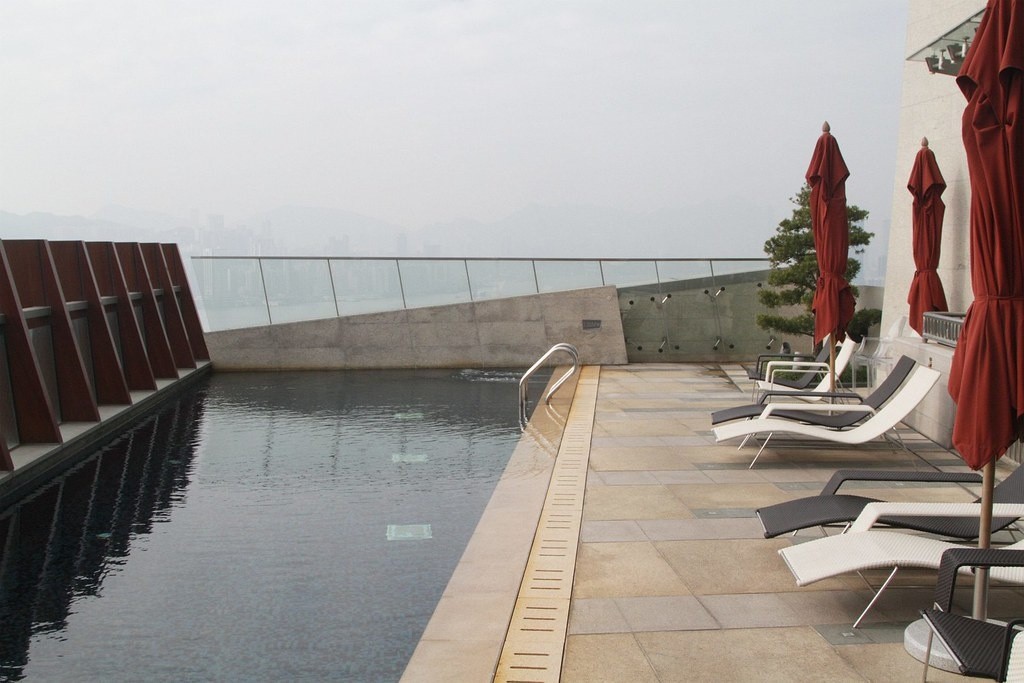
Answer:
[756,338,856,404]
[741,333,839,404]
[711,365,942,472]
[711,355,917,449]
[753,461,1024,683]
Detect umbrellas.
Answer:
[804,121,850,405]
[947,1,1024,624]
[907,137,947,344]
